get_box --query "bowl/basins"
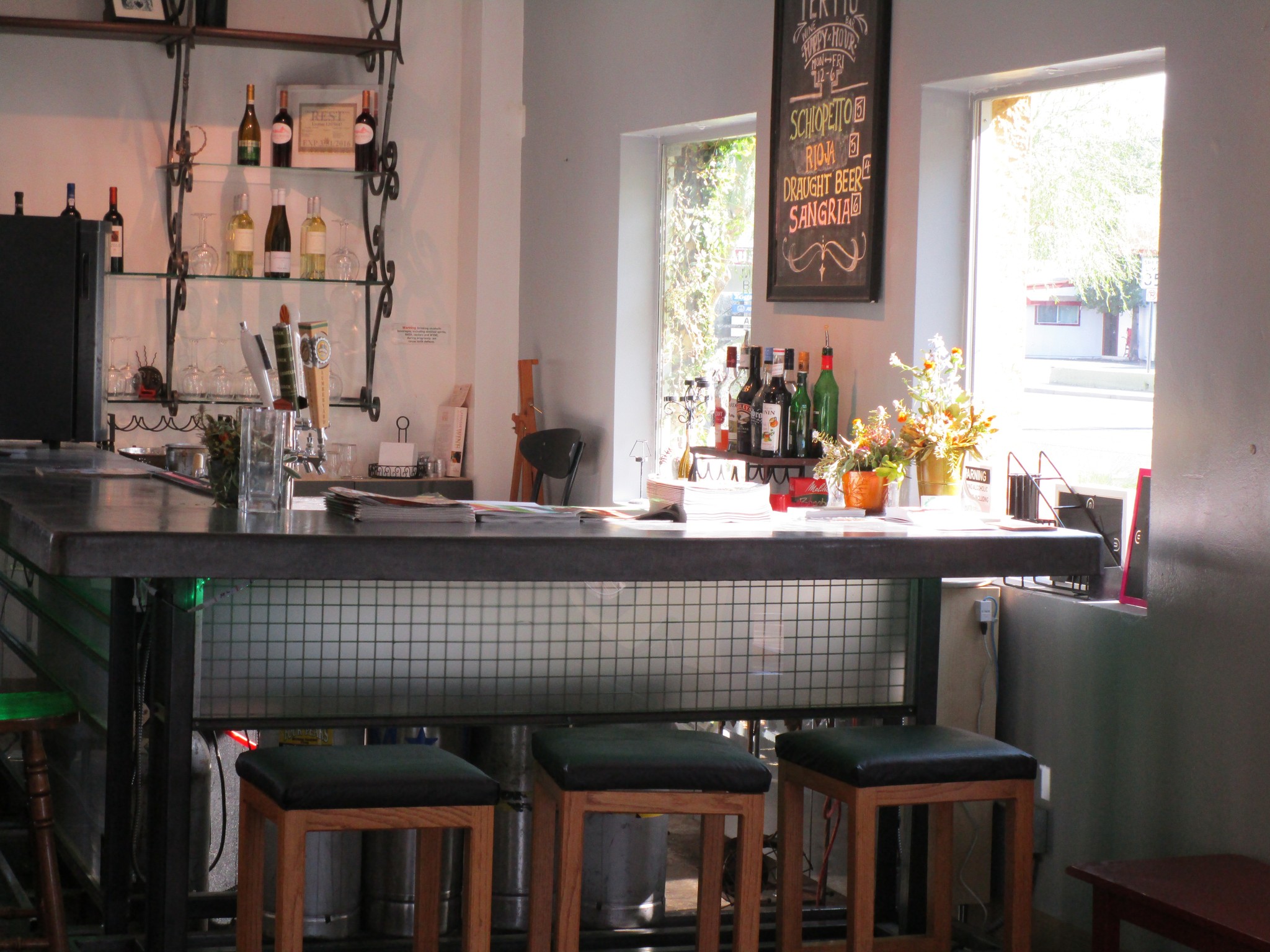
[117,444,211,478]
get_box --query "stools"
[527,723,772,952]
[237,742,499,952]
[0,679,79,952]
[771,725,1038,952]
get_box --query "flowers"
[813,333,998,487]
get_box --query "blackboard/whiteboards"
[765,0,891,303]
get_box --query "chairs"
[518,427,586,505]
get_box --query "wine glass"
[106,333,343,403]
[188,213,219,276]
[327,219,360,280]
[324,442,357,478]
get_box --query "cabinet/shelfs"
[0,1,406,420]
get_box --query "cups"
[427,456,445,479]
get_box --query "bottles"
[226,194,254,276]
[264,187,291,278]
[14,191,23,215]
[195,0,227,28]
[60,183,81,219]
[103,186,123,273]
[272,90,294,167]
[237,84,262,166]
[714,330,839,459]
[354,90,376,172]
[300,197,326,279]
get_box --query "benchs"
[1065,852,1270,952]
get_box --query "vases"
[841,471,890,516]
[916,449,962,498]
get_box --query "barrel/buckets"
[257,723,670,938]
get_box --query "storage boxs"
[434,401,468,477]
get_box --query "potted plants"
[202,414,301,508]
